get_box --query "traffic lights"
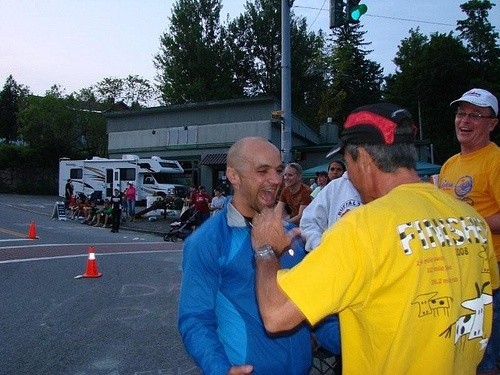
[346,0,367,23]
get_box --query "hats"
[450,88,498,117]
[325,103,417,159]
[129,182,133,185]
[316,171,328,177]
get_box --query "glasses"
[454,111,495,120]
[285,163,301,174]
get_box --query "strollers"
[163,206,203,244]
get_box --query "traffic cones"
[82,245,103,278]
[26,220,40,239]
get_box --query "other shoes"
[69,214,113,228]
[110,228,119,233]
[129,216,136,223]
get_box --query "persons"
[187,185,227,228]
[177,136,341,375]
[64,179,73,210]
[124,182,137,222]
[250,89,500,375]
[67,188,122,232]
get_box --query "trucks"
[59,155,186,205]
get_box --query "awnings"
[199,153,228,165]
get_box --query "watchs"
[254,244,275,258]
